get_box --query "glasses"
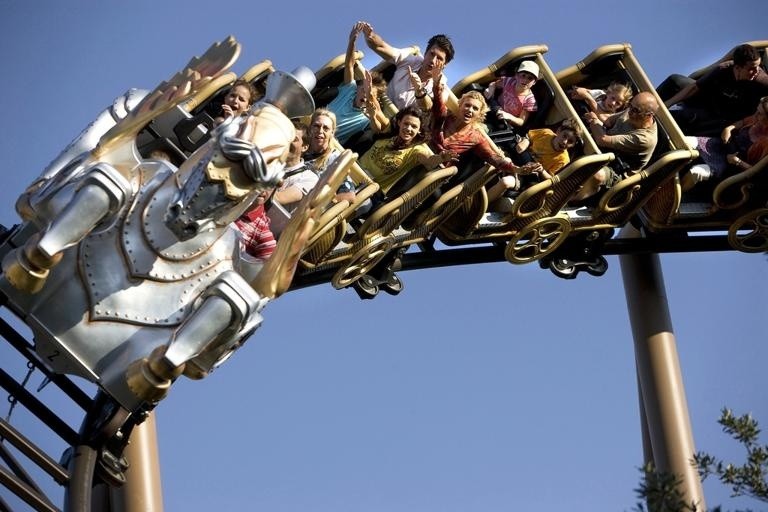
[628,103,650,114]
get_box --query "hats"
[516,60,541,79]
